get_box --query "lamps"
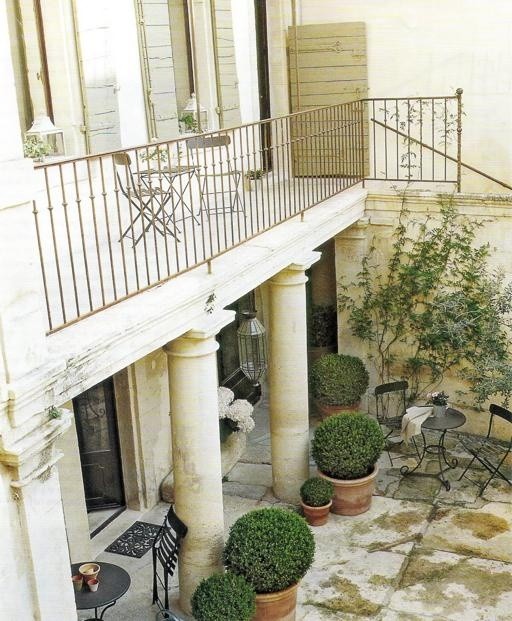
[237,311,268,387]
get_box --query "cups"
[71,563,101,592]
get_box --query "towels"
[400,406,433,446]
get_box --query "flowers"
[428,391,448,404]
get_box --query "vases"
[433,405,447,418]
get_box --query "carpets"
[105,521,172,559]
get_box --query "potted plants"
[311,408,384,516]
[306,307,340,360]
[300,479,336,524]
[222,507,317,621]
[311,350,369,419]
[248,170,264,191]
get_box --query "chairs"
[375,381,421,466]
[112,153,181,248]
[151,504,187,615]
[187,136,247,219]
[457,404,512,497]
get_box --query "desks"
[71,561,131,621]
[134,166,200,233]
[400,407,466,491]
